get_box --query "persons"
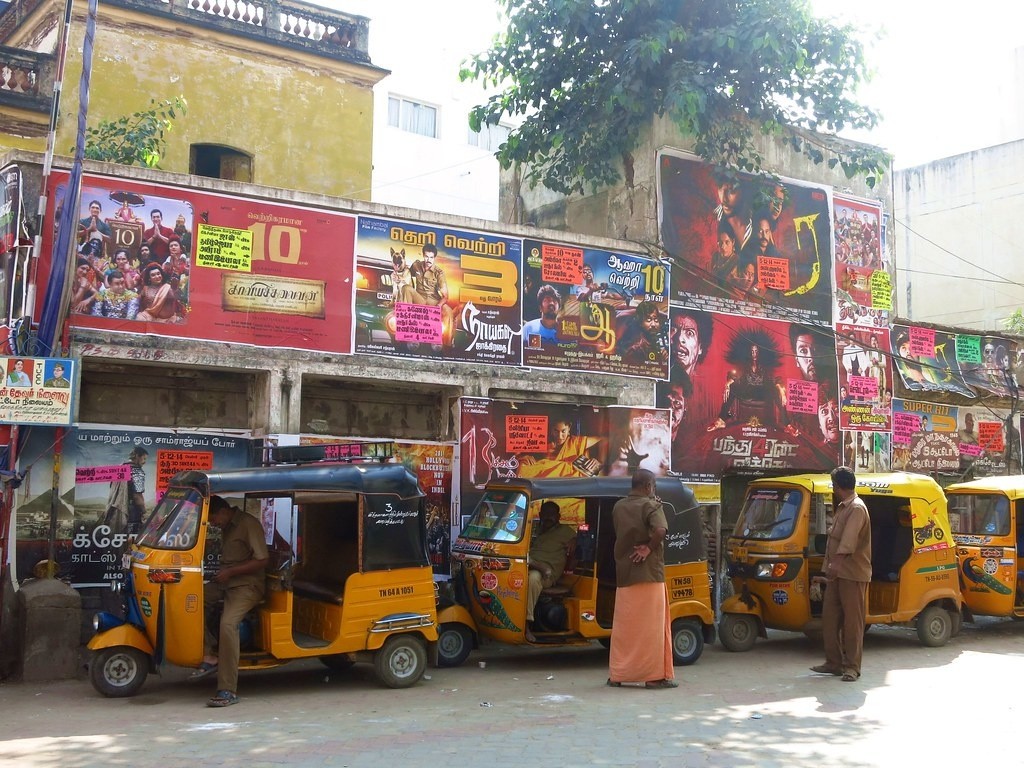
[71,200,189,323]
[841,332,892,403]
[607,469,679,688]
[110,447,148,533]
[710,176,787,304]
[188,495,269,706]
[394,243,453,349]
[958,414,978,444]
[609,411,669,475]
[834,209,880,270]
[526,502,578,642]
[810,467,872,681]
[516,414,608,524]
[894,332,1019,388]
[658,308,840,444]
[843,431,887,468]
[523,262,669,363]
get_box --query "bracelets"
[437,304,440,306]
[80,286,85,289]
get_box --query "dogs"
[389,248,415,306]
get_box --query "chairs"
[534,543,576,633]
[210,545,272,648]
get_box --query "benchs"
[563,566,592,600]
[872,526,914,614]
[293,579,357,643]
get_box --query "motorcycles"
[944,475,1024,627]
[425,473,718,667]
[87,441,439,699]
[716,469,964,651]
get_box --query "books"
[573,455,595,477]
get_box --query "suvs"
[355,255,468,357]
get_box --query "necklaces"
[147,287,159,302]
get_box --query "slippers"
[809,663,844,676]
[206,690,240,706]
[189,661,218,678]
[645,678,679,689]
[842,668,858,681]
[606,677,621,687]
